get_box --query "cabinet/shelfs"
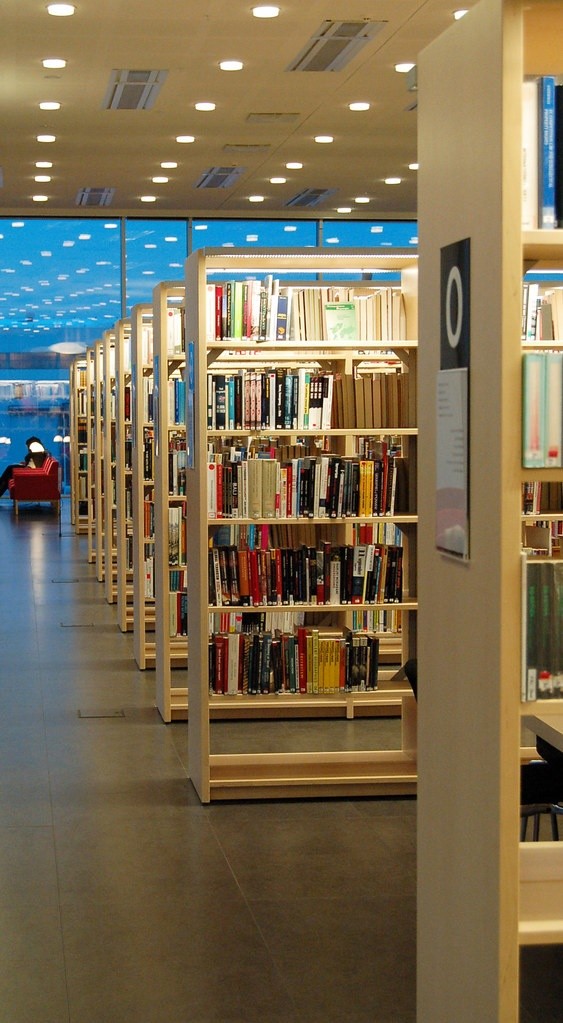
[70,0,563,1023]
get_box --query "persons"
[0,437,46,498]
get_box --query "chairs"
[8,456,61,515]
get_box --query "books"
[521,482,563,704]
[523,281,563,468]
[208,278,409,694]
[524,76,563,228]
[77,301,188,638]
